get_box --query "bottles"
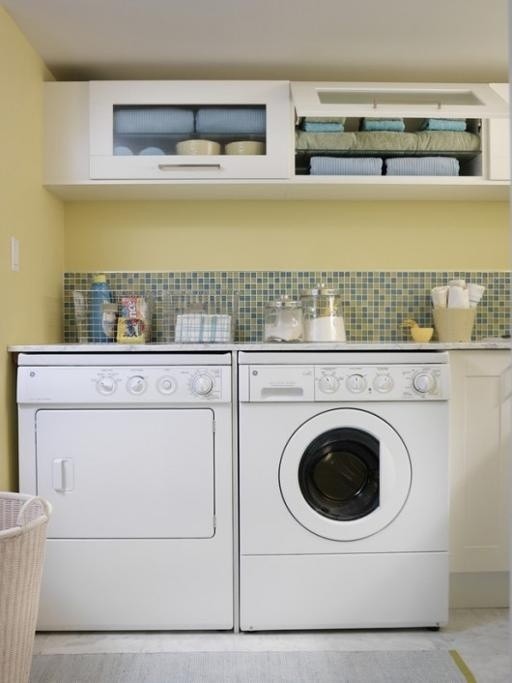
[262,281,346,344]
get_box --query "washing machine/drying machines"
[6,340,238,636]
[236,340,455,633]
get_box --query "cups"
[432,308,476,343]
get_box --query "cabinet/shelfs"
[448,347,511,575]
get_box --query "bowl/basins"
[175,138,265,156]
[410,327,435,343]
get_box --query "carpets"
[28,651,477,683]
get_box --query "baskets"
[0,491,52,683]
